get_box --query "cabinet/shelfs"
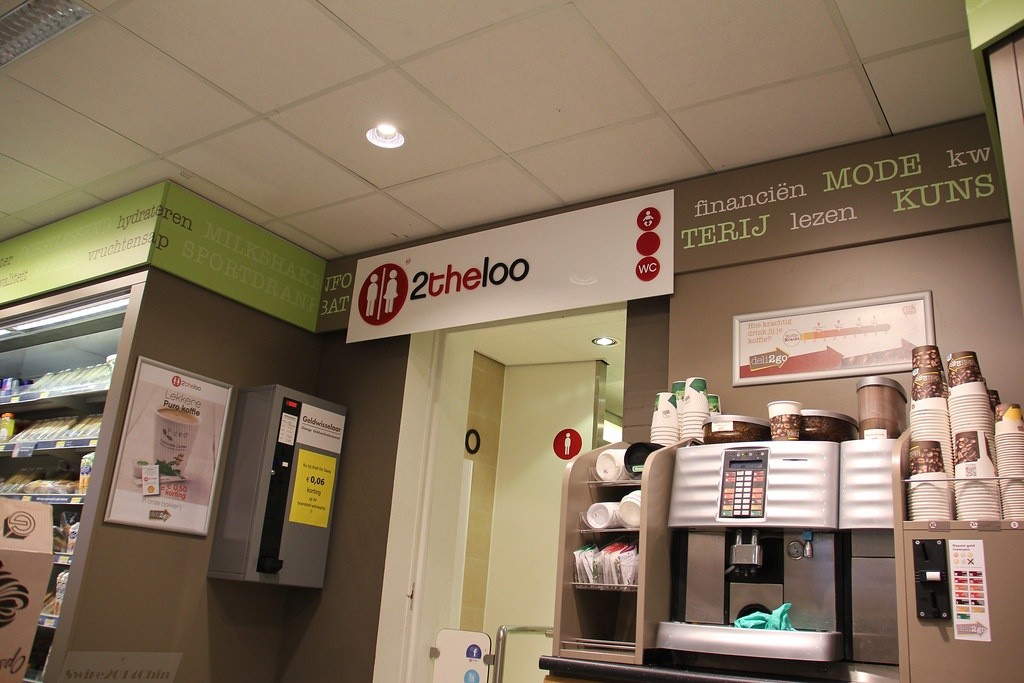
[0,295,132,683]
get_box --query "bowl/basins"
[800,409,859,442]
[702,415,769,443]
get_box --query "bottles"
[0,376,33,402]
[0,413,15,448]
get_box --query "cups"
[907,345,1024,520]
[134,461,149,478]
[105,353,118,377]
[156,408,199,477]
[649,377,722,446]
[767,400,801,441]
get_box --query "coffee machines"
[653,435,900,683]
